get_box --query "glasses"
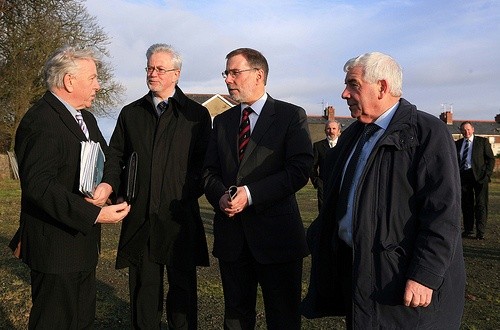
[222,67,258,78]
[144,67,175,74]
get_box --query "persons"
[8,45,130,330]
[310,119,343,215]
[105,44,212,329]
[199,48,316,330]
[454,121,496,241]
[298,51,465,330]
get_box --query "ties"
[75,112,89,141]
[460,140,470,170]
[337,123,380,222]
[238,107,254,164]
[158,101,167,116]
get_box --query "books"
[79,138,105,200]
